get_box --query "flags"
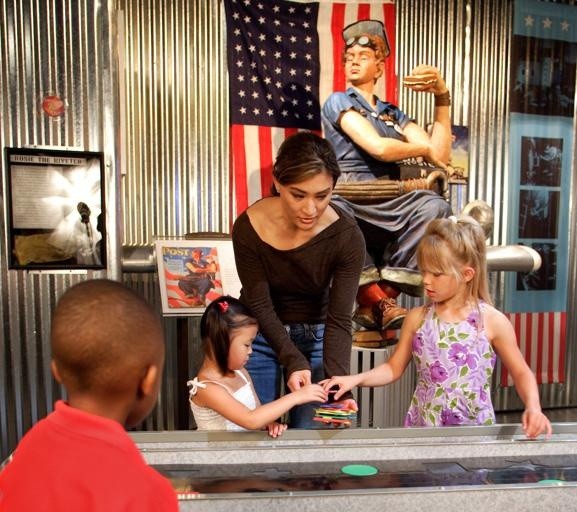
[221,0,399,221]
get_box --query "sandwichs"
[403,73,438,90]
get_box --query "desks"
[121,422,577,512]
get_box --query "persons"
[320,31,459,333]
[0,276,180,511]
[177,248,216,305]
[73,202,100,266]
[314,212,554,439]
[188,296,327,438]
[229,136,367,430]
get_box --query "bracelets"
[432,91,451,107]
